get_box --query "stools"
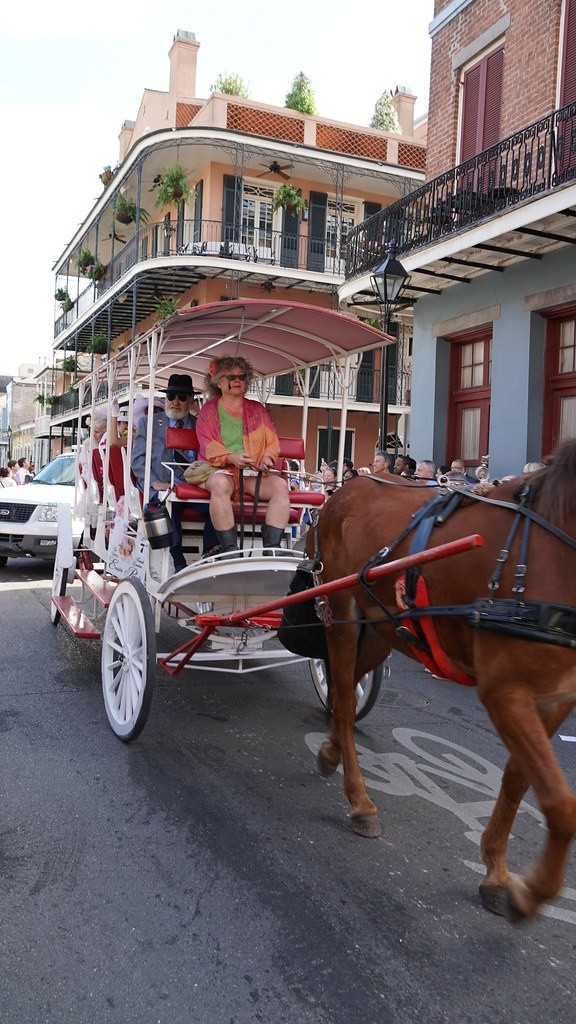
[487,187,519,199]
[425,215,453,229]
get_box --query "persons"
[0,466,17,566]
[524,463,545,472]
[110,394,165,448]
[289,458,370,516]
[7,458,35,485]
[131,374,220,572]
[79,405,108,483]
[372,452,480,485]
[99,406,130,460]
[195,355,294,561]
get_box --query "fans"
[256,160,296,181]
[99,232,127,244]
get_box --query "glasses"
[167,392,187,402]
[218,374,247,380]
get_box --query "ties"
[174,419,189,481]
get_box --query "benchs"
[159,426,327,572]
[98,444,126,511]
[74,454,90,520]
[87,447,103,517]
[122,443,161,521]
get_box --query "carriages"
[47,298,575,922]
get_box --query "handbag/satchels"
[183,461,222,484]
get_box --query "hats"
[133,395,165,428]
[117,406,129,422]
[158,374,201,394]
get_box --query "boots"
[215,524,240,560]
[261,523,294,558]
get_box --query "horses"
[305,436,575,926]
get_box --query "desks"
[438,189,487,215]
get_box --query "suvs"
[0,450,76,568]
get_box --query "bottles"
[143,501,174,550]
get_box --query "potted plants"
[60,354,81,375]
[272,185,307,218]
[32,392,59,406]
[85,332,116,356]
[153,165,200,209]
[112,193,151,225]
[98,164,114,184]
[54,288,74,312]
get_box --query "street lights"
[368,238,412,453]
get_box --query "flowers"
[75,247,108,280]
